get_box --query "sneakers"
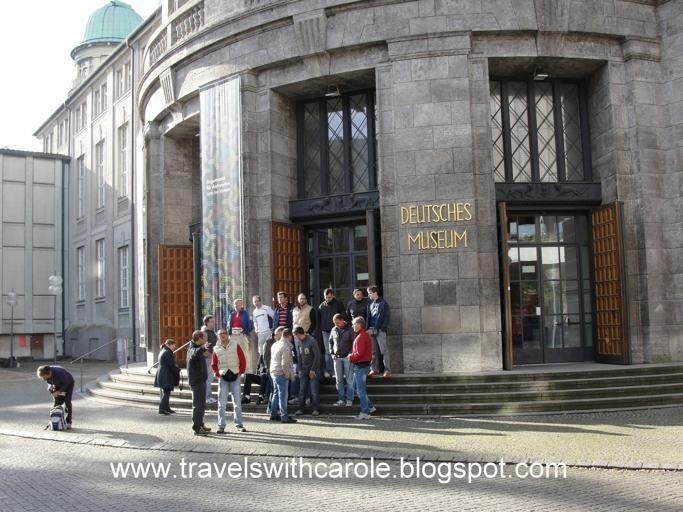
[383,372,390,377]
[324,372,346,378]
[367,370,378,377]
[237,426,245,432]
[66,423,70,429]
[241,397,267,405]
[194,424,223,436]
[209,398,217,403]
[288,399,318,416]
[205,400,213,404]
[267,409,297,422]
[332,400,375,421]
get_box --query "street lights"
[5,287,17,369]
[46,270,63,366]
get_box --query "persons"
[327,310,356,408]
[261,325,285,414]
[251,294,274,358]
[292,326,322,419]
[270,290,296,336]
[36,364,74,430]
[153,337,180,414]
[185,329,211,436]
[239,353,271,404]
[209,327,247,434]
[366,282,392,380]
[268,328,297,424]
[345,286,368,327]
[199,314,217,405]
[290,291,317,406]
[224,298,250,337]
[346,314,378,422]
[316,287,345,380]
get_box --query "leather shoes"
[159,410,169,414]
[167,408,173,413]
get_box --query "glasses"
[218,333,225,335]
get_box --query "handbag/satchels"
[44,405,67,431]
[367,326,378,337]
[174,368,183,391]
